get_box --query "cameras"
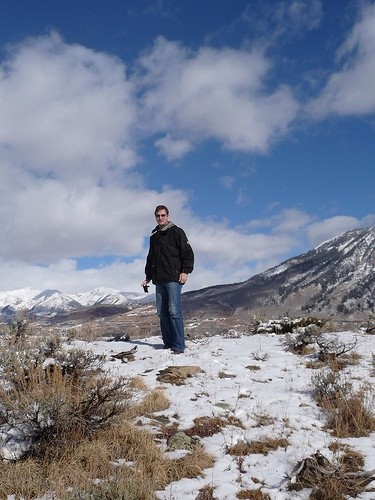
[143,285,149,293]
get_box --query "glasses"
[156,214,167,217]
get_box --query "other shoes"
[171,350,181,354]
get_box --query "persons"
[142,205,194,354]
